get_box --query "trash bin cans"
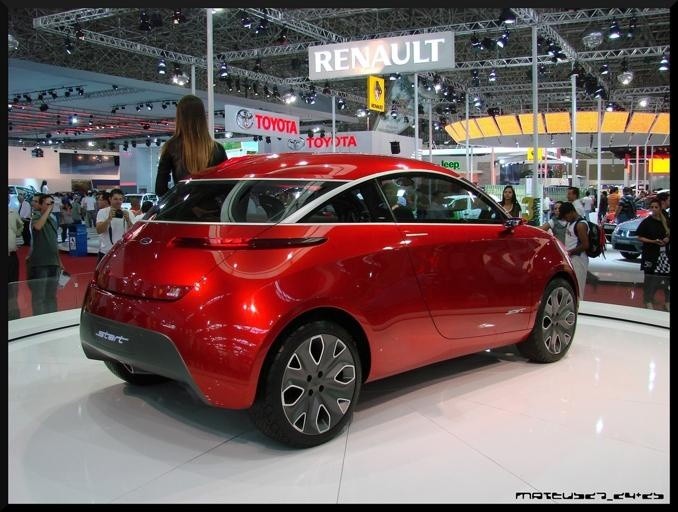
[68,224,88,256]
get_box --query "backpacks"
[575,218,607,258]
[622,202,636,219]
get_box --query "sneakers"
[646,303,654,309]
[663,301,670,310]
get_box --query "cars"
[118,192,158,210]
[602,182,669,259]
[78,151,579,450]
[444,193,502,220]
[7,184,44,213]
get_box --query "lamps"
[659,64,669,71]
[660,52,669,64]
[9,8,638,157]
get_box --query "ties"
[18,204,22,216]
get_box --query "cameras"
[112,209,123,218]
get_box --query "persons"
[155,95,227,197]
[7,173,669,322]
